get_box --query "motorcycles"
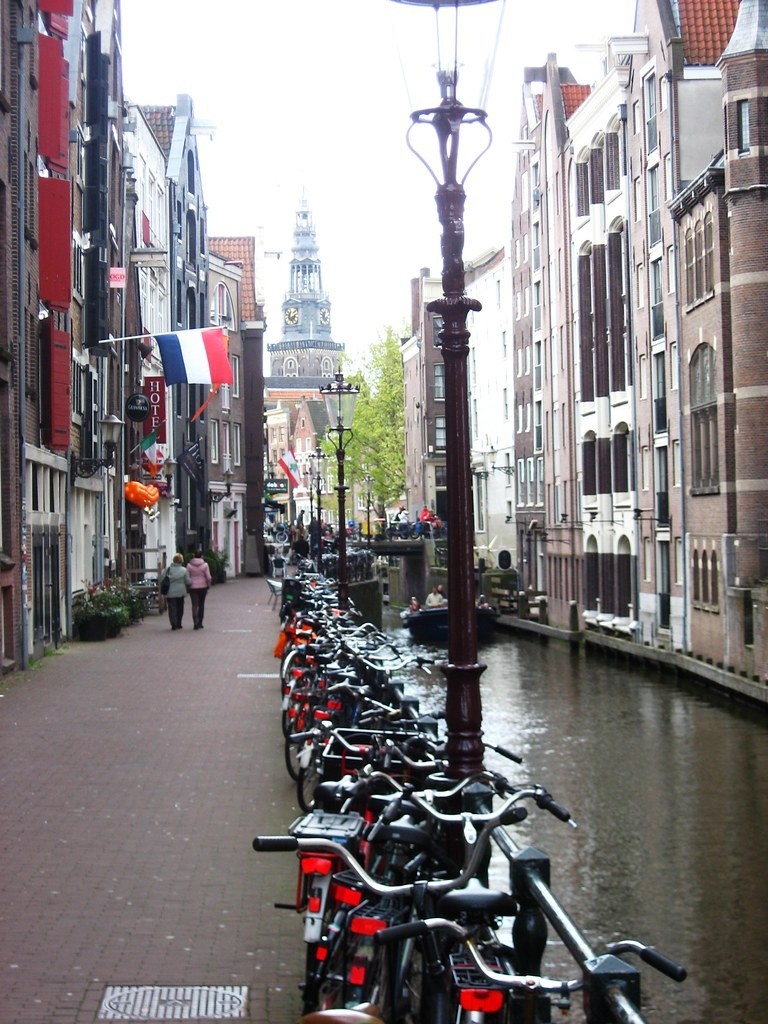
[276,523,291,542]
[386,521,447,539]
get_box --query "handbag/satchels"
[160,567,169,595]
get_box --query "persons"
[296,510,335,538]
[292,535,309,563]
[162,553,192,630]
[477,594,490,609]
[393,506,441,537]
[186,551,212,630]
[409,584,447,613]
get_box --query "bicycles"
[251,559,692,1024]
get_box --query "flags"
[140,427,157,478]
[279,448,301,488]
[153,329,233,387]
[177,440,202,482]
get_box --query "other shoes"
[177,626,183,628]
[198,620,203,628]
[193,621,198,629]
[172,624,177,630]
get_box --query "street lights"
[319,374,361,610]
[361,472,375,542]
[307,446,329,559]
[395,0,493,778]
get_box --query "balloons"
[126,481,159,509]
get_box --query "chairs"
[265,578,282,611]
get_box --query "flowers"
[71,577,149,627]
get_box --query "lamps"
[505,508,675,542]
[158,457,178,492]
[70,414,126,483]
[208,470,234,505]
[138,342,154,357]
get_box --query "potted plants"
[185,549,227,586]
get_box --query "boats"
[403,608,495,648]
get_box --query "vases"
[77,616,121,640]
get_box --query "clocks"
[320,308,330,324]
[284,306,298,325]
